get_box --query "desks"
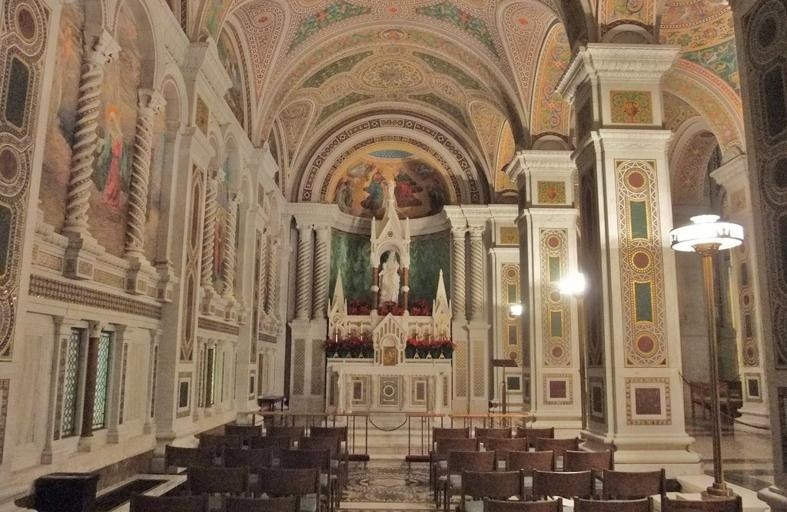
[258,396,285,418]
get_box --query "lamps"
[511,297,528,315]
[559,268,587,300]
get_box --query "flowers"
[324,336,372,350]
[408,336,455,350]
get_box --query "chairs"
[131,425,351,512]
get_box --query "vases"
[325,348,373,358]
[405,348,452,358]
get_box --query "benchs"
[690,382,733,423]
